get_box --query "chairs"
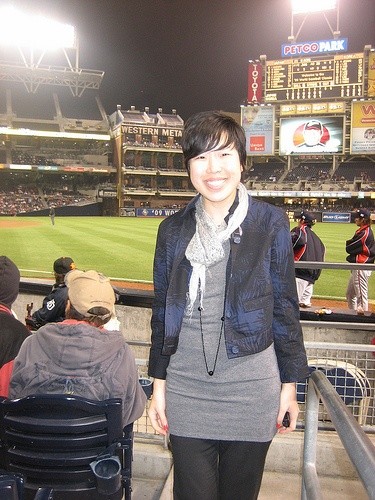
[0,393,131,499]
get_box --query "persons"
[149,110,310,500]
[0,256,32,398]
[33,256,75,331]
[8,269,147,430]
[346,208,375,312]
[291,212,325,306]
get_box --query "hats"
[348,208,371,220]
[0,255,20,305]
[294,211,317,228]
[54,256,75,274]
[64,269,115,319]
